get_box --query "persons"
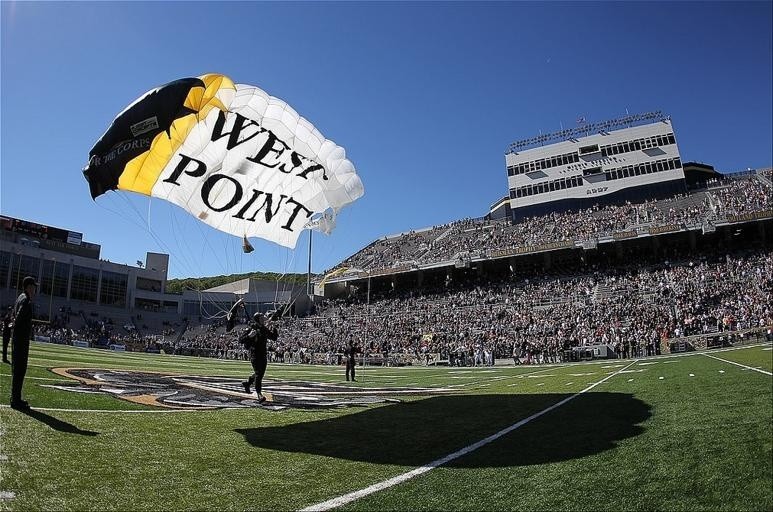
[1,305,13,365]
[311,283,772,364]
[28,308,312,363]
[312,171,771,284]
[235,311,279,404]
[9,274,39,411]
[340,341,364,384]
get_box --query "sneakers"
[9,398,28,409]
[242,381,266,405]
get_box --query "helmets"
[253,312,264,323]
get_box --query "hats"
[22,276,40,288]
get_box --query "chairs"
[0,290,212,343]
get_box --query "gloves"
[247,329,257,339]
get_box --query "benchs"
[345,187,710,272]
